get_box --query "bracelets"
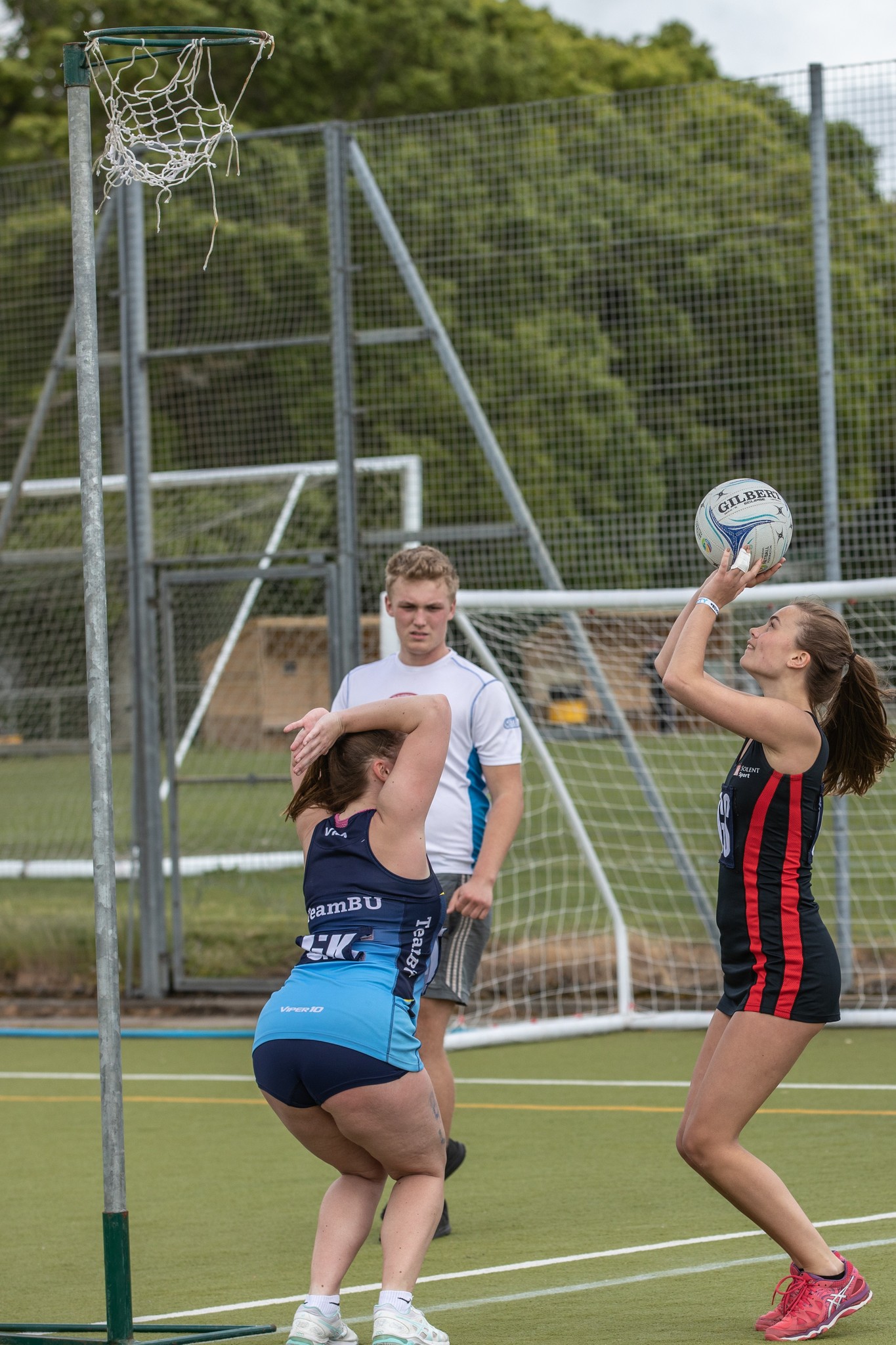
[696,598,719,616]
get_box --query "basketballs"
[694,478,793,575]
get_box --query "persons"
[656,544,896,1345]
[250,695,452,1345]
[330,546,526,1241]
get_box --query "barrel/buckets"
[550,683,591,724]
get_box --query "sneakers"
[285,1305,359,1345]
[755,1250,846,1331]
[371,1304,450,1345]
[765,1261,873,1342]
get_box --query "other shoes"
[380,1200,450,1240]
[444,1137,467,1179]
